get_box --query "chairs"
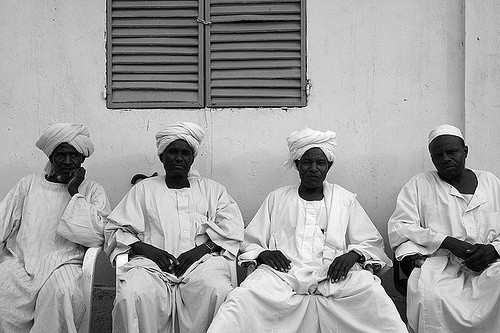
[79,246,408,333]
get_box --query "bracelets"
[349,249,364,264]
[204,242,215,253]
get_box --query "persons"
[205,129,408,333]
[104,122,244,333]
[388,124,500,333]
[0,123,112,333]
